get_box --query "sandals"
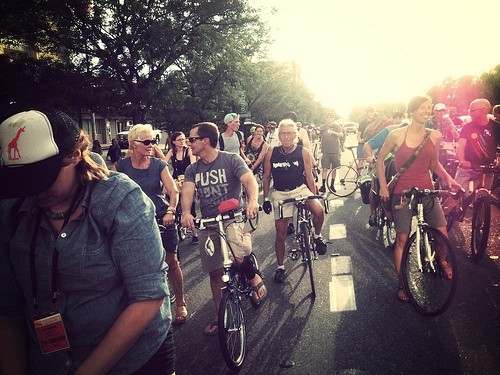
[175,302,187,323]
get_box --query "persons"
[151,131,200,245]
[493,105,500,122]
[449,107,463,125]
[181,122,267,336]
[79,131,107,169]
[218,113,277,175]
[441,99,500,212]
[92,140,102,155]
[112,124,188,321]
[107,139,121,165]
[0,108,175,375]
[263,118,327,282]
[377,95,462,303]
[356,107,410,228]
[317,112,343,193]
[271,111,321,235]
[425,103,459,163]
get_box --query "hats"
[0,108,81,200]
[224,113,240,124]
[250,126,256,134]
[450,107,457,111]
[282,112,298,122]
[433,103,446,112]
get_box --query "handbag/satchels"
[377,176,397,212]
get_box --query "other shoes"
[330,186,336,192]
[369,214,377,226]
[192,235,199,244]
[274,268,285,282]
[288,223,293,234]
[319,186,326,192]
[439,257,454,279]
[397,287,410,301]
[313,237,326,255]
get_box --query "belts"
[274,182,304,191]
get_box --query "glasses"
[136,139,157,145]
[177,139,184,141]
[469,109,481,113]
[188,136,205,141]
[327,117,332,118]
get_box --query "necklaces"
[46,186,86,221]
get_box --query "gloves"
[263,201,272,214]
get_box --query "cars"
[344,123,357,134]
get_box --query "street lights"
[447,94,456,107]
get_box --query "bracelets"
[167,207,176,213]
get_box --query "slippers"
[203,320,219,336]
[251,272,266,302]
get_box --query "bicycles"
[156,192,262,304]
[277,194,327,297]
[362,156,500,317]
[325,145,372,197]
[191,199,265,371]
